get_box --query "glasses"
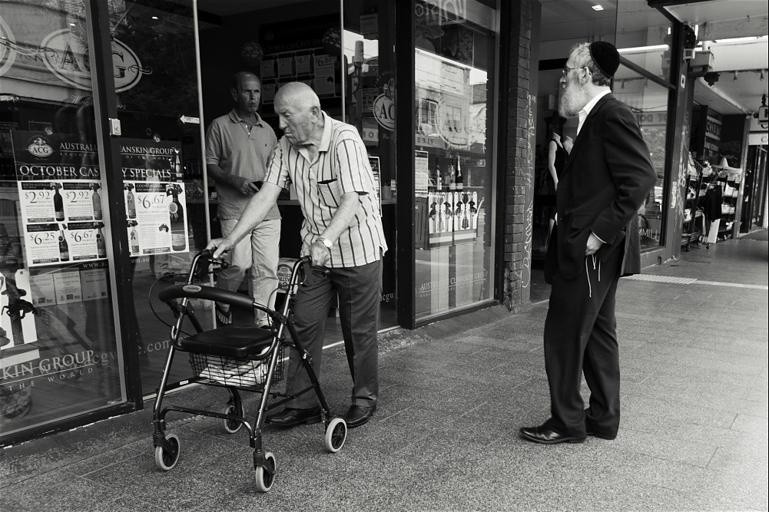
[562,67,583,76]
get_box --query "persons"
[549,131,575,235]
[205,82,389,426]
[205,71,283,382]
[519,39,657,444]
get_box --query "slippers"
[215,301,234,326]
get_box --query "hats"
[588,39,622,79]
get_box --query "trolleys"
[0,253,106,422]
[153,248,348,493]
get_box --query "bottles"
[170,187,186,252]
[58,230,70,263]
[96,224,106,259]
[127,187,137,219]
[433,153,463,192]
[92,184,103,221]
[130,224,139,253]
[53,186,66,221]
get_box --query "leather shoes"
[343,400,377,428]
[583,406,618,440]
[519,418,586,444]
[262,402,323,428]
